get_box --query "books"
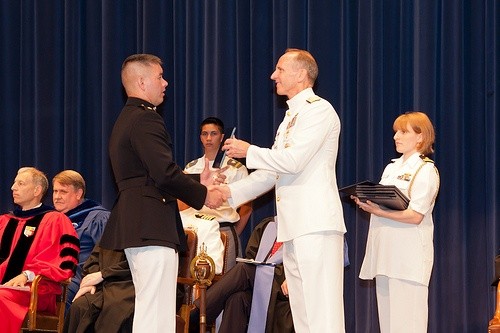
[212,126,236,169]
[338,179,410,210]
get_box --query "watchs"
[24,272,27,276]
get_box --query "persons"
[176,198,225,305]
[62,233,185,333]
[0,167,80,333]
[188,216,295,333]
[183,117,252,274]
[98,53,230,333]
[350,111,441,333]
[52,170,111,318]
[206,48,347,333]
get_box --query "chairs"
[21,274,68,333]
[175,228,228,333]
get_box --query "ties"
[272,241,282,255]
